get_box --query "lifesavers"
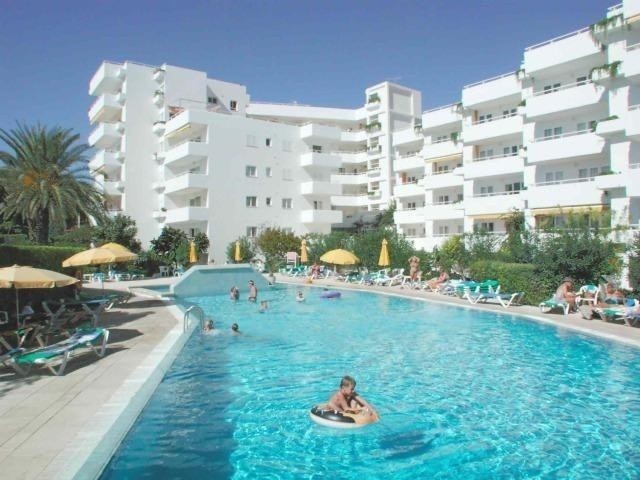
[310,403,378,430]
[320,292,340,298]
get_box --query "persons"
[605,282,624,298]
[247,280,257,303]
[204,319,213,332]
[596,299,640,315]
[431,272,447,284]
[229,323,242,335]
[316,262,322,277]
[260,301,269,313]
[230,285,240,301]
[311,262,316,275]
[322,376,380,422]
[22,299,34,321]
[408,255,420,280]
[296,291,304,302]
[554,276,576,312]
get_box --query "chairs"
[541,284,639,325]
[278,264,423,288]
[0,292,132,376]
[411,275,522,308]
[159,264,182,277]
[83,271,146,281]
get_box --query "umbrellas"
[0,263,81,328]
[320,248,360,264]
[301,239,308,265]
[189,241,197,263]
[61,244,139,295]
[101,241,130,270]
[377,238,391,268]
[235,237,242,264]
